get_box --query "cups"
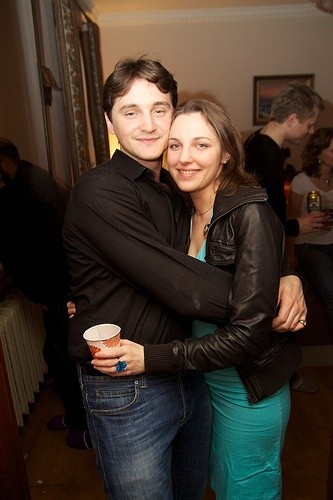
[83,324,121,359]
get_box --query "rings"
[300,320,306,325]
[116,361,127,373]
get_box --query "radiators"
[0,284,49,428]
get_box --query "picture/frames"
[254,73,314,124]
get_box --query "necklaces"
[196,202,214,215]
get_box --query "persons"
[289,128,333,345]
[243,83,326,394]
[62,53,308,500]
[68,99,307,500]
[0,138,93,449]
